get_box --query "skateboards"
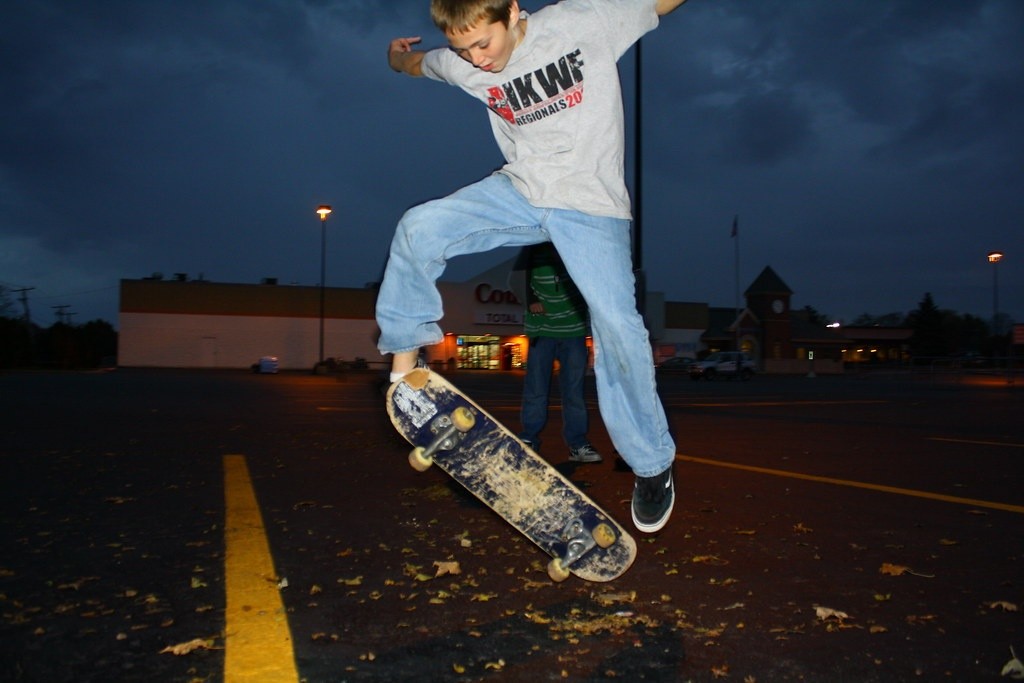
[386,368,638,583]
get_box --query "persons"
[506,232,610,466]
[374,1,679,533]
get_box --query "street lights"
[986,251,1005,374]
[315,204,333,359]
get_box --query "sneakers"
[569,444,601,462]
[632,462,677,532]
[522,439,535,450]
[384,357,431,395]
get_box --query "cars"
[252,356,280,374]
[655,356,697,374]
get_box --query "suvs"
[687,350,760,383]
